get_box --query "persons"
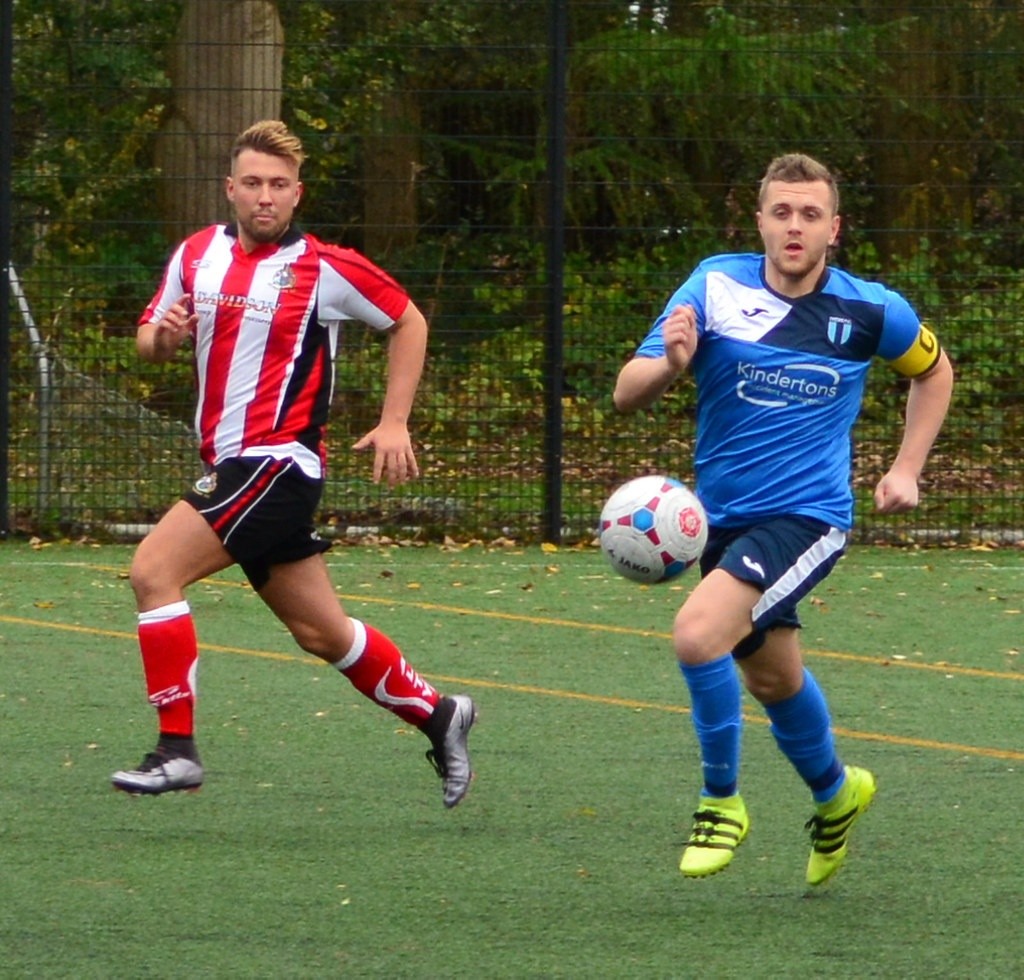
[112,118,476,808]
[614,153,957,885]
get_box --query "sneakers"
[110,734,204,796]
[419,694,476,807]
[805,764,877,886]
[679,791,749,879]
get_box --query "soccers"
[598,475,707,584]
